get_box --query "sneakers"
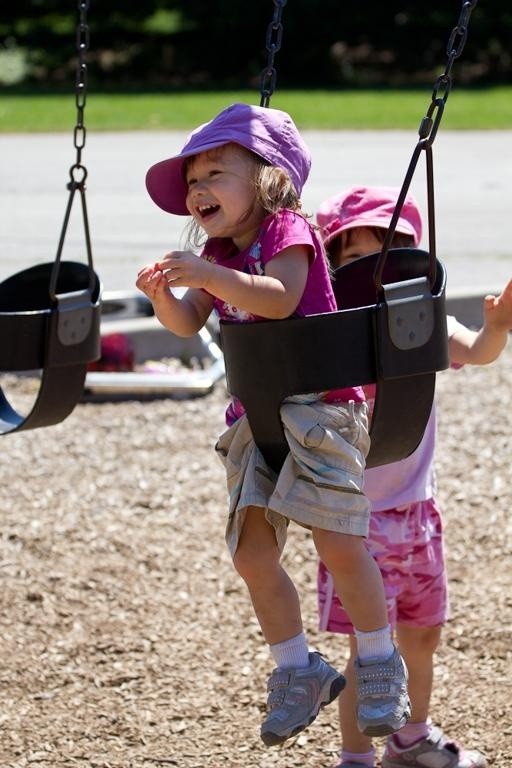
[353,641,413,737]
[381,725,487,768]
[260,652,347,747]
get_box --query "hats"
[145,103,313,218]
[314,188,423,251]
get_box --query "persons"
[271,185,510,766]
[129,104,415,745]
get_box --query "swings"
[219,1,477,479]
[1,1,102,436]
